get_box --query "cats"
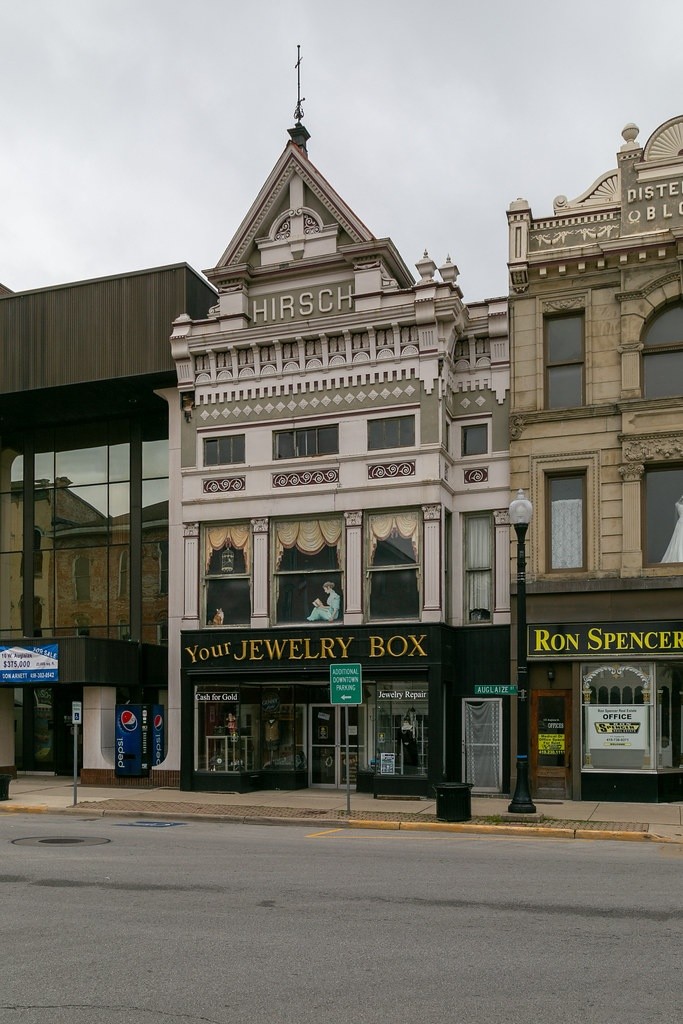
[214,608,224,625]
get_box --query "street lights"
[507,488,538,814]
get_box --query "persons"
[263,719,278,766]
[396,721,421,775]
[660,495,683,563]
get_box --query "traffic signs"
[330,664,363,704]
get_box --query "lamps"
[547,665,556,688]
